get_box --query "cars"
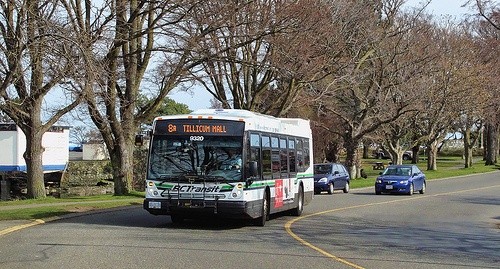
[314,163,350,194]
[376,147,413,160]
[374,164,427,196]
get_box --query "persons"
[220,150,242,170]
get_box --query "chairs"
[396,168,401,174]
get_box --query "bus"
[134,109,313,227]
